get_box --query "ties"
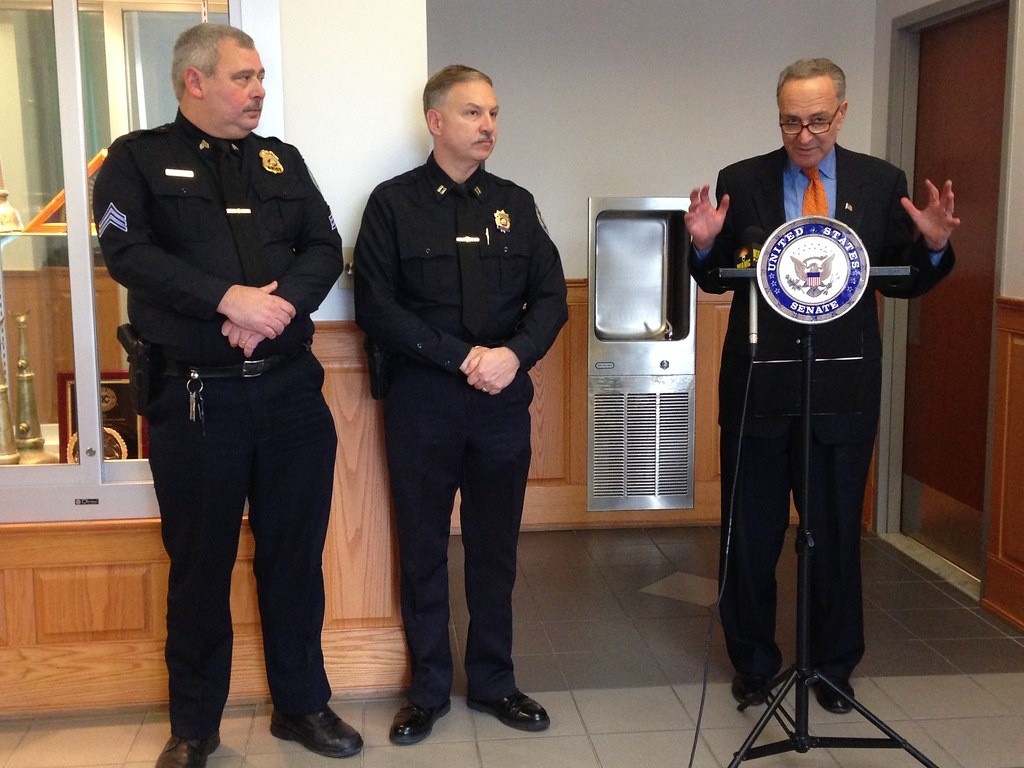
[454,183,493,337]
[219,141,269,287]
[800,166,828,218]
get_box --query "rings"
[483,387,488,392]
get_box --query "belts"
[158,356,280,384]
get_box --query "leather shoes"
[730,651,782,705]
[466,691,550,731]
[154,729,220,768]
[388,699,451,744]
[815,680,856,712]
[270,707,364,757]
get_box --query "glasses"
[779,106,840,134]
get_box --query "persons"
[352,60,571,745]
[92,23,364,767]
[683,53,961,714]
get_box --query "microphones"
[732,225,765,358]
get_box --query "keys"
[188,391,206,436]
